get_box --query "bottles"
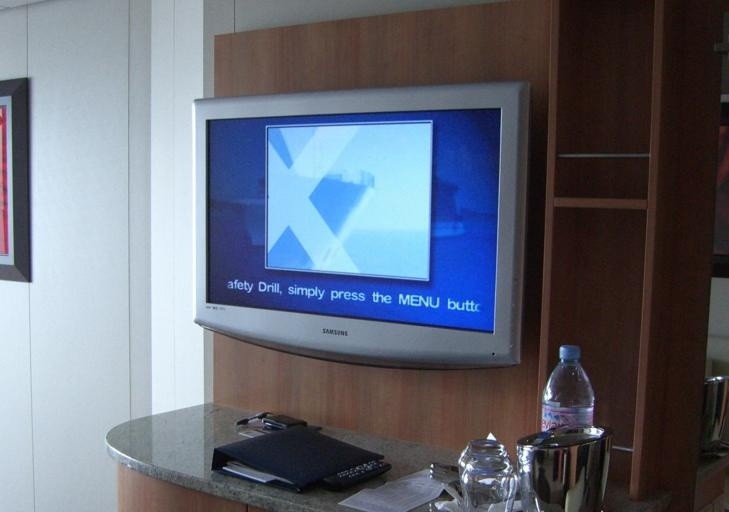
[541,346,595,432]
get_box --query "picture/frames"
[0,78,34,283]
[710,104,728,277]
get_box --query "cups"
[458,439,515,512]
[460,454,517,512]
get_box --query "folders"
[212,424,384,494]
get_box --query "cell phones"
[261,414,308,432]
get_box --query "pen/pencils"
[236,412,268,425]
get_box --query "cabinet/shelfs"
[540,1,664,499]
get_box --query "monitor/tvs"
[192,80,532,371]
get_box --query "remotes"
[322,459,393,492]
